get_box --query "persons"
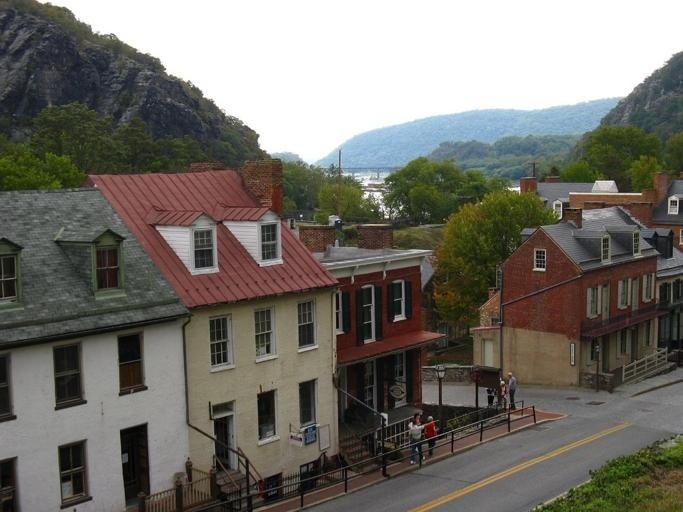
[408,413,439,465]
[487,372,516,412]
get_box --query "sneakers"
[423,456,425,460]
[410,461,416,464]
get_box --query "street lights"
[595,343,601,393]
[435,365,447,438]
